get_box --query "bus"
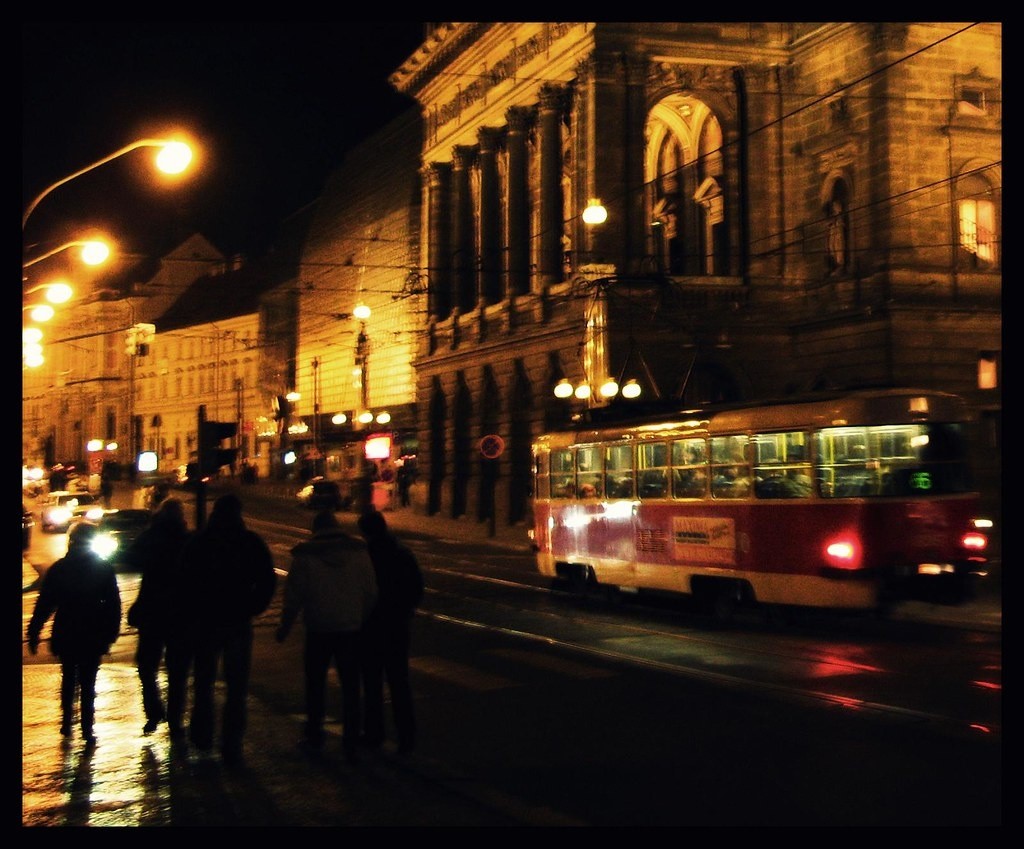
[527,386,1000,629]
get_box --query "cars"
[22,505,40,550]
[40,489,107,532]
[98,508,154,564]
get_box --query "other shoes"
[387,750,418,770]
[144,711,165,734]
[83,733,97,742]
[61,725,71,735]
[298,737,323,756]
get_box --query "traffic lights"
[124,321,157,362]
[196,401,239,476]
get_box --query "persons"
[273,507,379,753]
[126,495,279,763]
[398,459,416,507]
[355,511,425,756]
[550,435,933,499]
[26,524,120,743]
